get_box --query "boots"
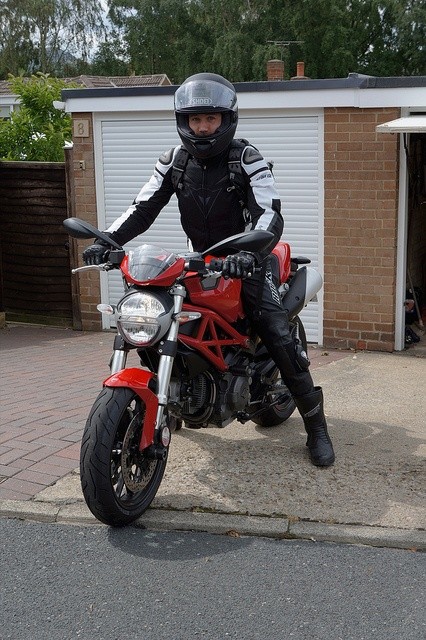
[292,386,335,467]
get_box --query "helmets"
[173,72,239,161]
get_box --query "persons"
[81,72,335,466]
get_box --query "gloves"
[221,252,256,281]
[82,241,110,266]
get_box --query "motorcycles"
[61,215,323,527]
